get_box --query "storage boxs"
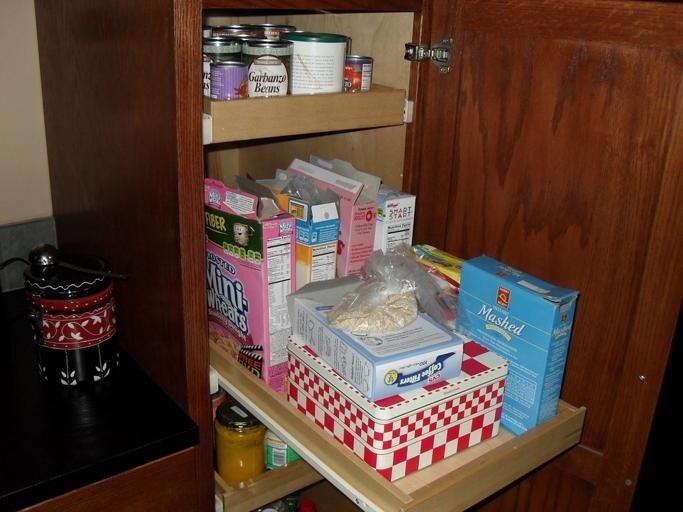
[409,242,463,315]
[205,160,417,390]
[453,253,580,439]
[287,282,508,482]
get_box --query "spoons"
[27,241,131,282]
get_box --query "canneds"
[345,55,373,92]
[214,398,267,488]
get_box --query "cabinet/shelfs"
[33,0,683,511]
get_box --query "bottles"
[205,382,298,485]
[20,252,122,396]
